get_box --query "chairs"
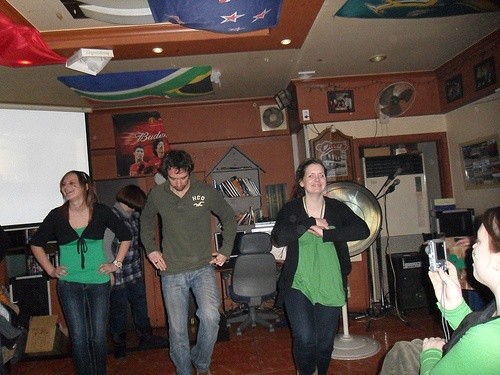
[226,232,280,336]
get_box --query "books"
[215,205,260,228]
[213,176,261,198]
[27,250,59,275]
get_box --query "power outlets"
[303,110,310,121]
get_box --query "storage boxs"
[430,208,477,239]
[25,315,68,358]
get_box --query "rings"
[155,261,158,264]
[216,258,218,261]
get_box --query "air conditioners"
[361,152,430,308]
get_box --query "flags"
[333,0,500,19]
[56,64,215,105]
[147,0,283,34]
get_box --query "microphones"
[384,167,402,186]
[387,179,400,192]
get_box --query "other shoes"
[192,365,210,375]
[113,343,126,359]
[142,337,165,347]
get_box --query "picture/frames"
[473,55,496,92]
[444,73,464,104]
[327,90,355,113]
[456,132,500,190]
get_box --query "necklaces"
[304,195,325,219]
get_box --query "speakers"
[386,251,436,314]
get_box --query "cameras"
[425,239,448,272]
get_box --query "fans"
[323,181,382,360]
[374,81,416,124]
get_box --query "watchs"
[112,259,123,269]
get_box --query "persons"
[141,150,238,375]
[0,225,28,375]
[104,184,169,359]
[379,206,500,375]
[453,236,477,247]
[271,157,371,375]
[29,170,133,375]
[148,138,166,176]
[129,145,157,177]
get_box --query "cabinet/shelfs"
[205,144,266,230]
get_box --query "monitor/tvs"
[215,231,246,258]
